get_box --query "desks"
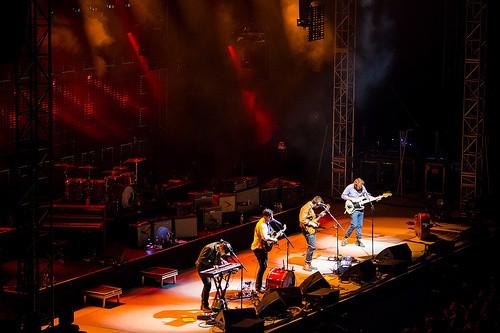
[141,268,177,287]
[81,285,122,308]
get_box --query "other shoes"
[201,305,212,313]
[356,239,366,247]
[256,289,265,294]
[303,262,312,271]
[341,237,347,246]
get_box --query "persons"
[195,241,230,313]
[341,178,381,247]
[299,196,326,271]
[251,208,284,294]
[402,271,500,333]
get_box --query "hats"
[222,241,231,255]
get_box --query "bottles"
[239,214,244,224]
[146,233,179,250]
[274,203,282,212]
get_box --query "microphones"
[272,218,281,225]
[318,202,326,206]
[358,191,366,197]
[220,239,230,246]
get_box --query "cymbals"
[113,167,129,170]
[78,166,97,169]
[54,164,77,168]
[125,158,146,162]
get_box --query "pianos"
[199,263,242,315]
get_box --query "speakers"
[340,243,412,283]
[214,271,339,333]
[126,177,303,249]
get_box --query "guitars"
[345,191,392,215]
[262,224,287,252]
[301,204,330,235]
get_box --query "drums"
[90,180,108,201]
[266,267,295,289]
[121,172,136,184]
[111,184,135,209]
[72,178,87,200]
[104,175,122,183]
